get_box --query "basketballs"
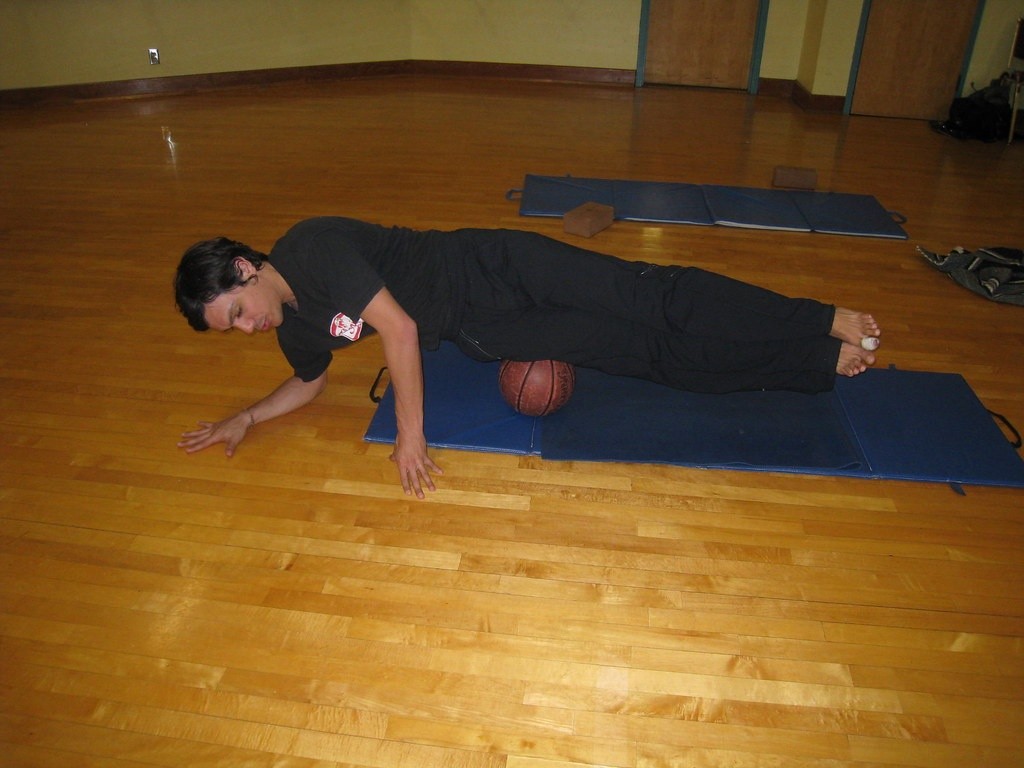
[497,358,577,417]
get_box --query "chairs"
[1001,17,1024,146]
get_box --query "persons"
[174,217,879,499]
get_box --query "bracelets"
[246,409,254,427]
